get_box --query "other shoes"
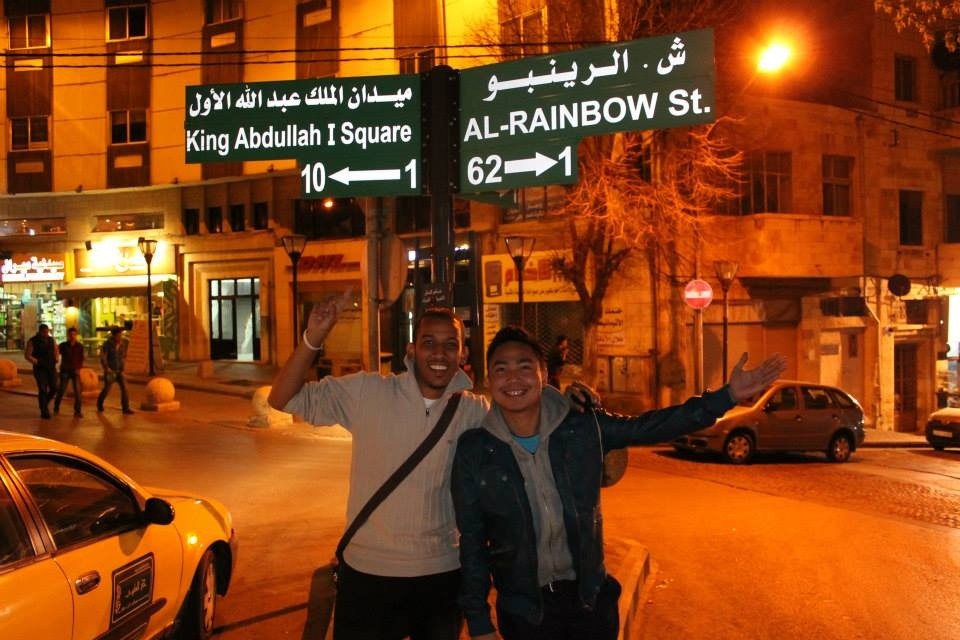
[40,411,51,418]
[74,405,81,413]
[53,400,58,411]
[96,402,104,412]
[122,408,135,415]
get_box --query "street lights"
[279,234,306,352]
[503,235,537,329]
[137,236,158,377]
[711,258,739,387]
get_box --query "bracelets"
[303,329,324,352]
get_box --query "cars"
[0,426,240,640]
[669,377,866,466]
[923,396,960,453]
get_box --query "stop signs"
[684,278,713,309]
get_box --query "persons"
[448,326,788,640]
[23,324,60,419]
[267,299,601,640]
[95,327,138,414]
[54,327,87,418]
[546,335,570,390]
